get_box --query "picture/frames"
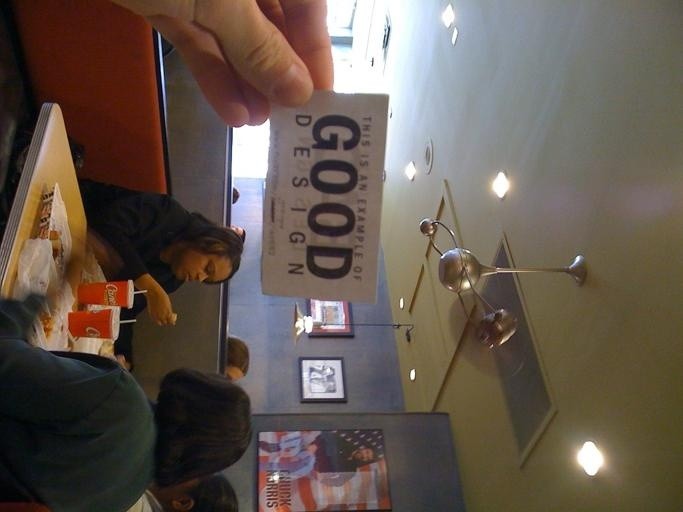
[304,298,354,338]
[255,429,392,512]
[297,356,347,404]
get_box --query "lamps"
[292,301,415,347]
[419,218,587,351]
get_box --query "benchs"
[0,0,171,197]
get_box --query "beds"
[0,102,114,367]
